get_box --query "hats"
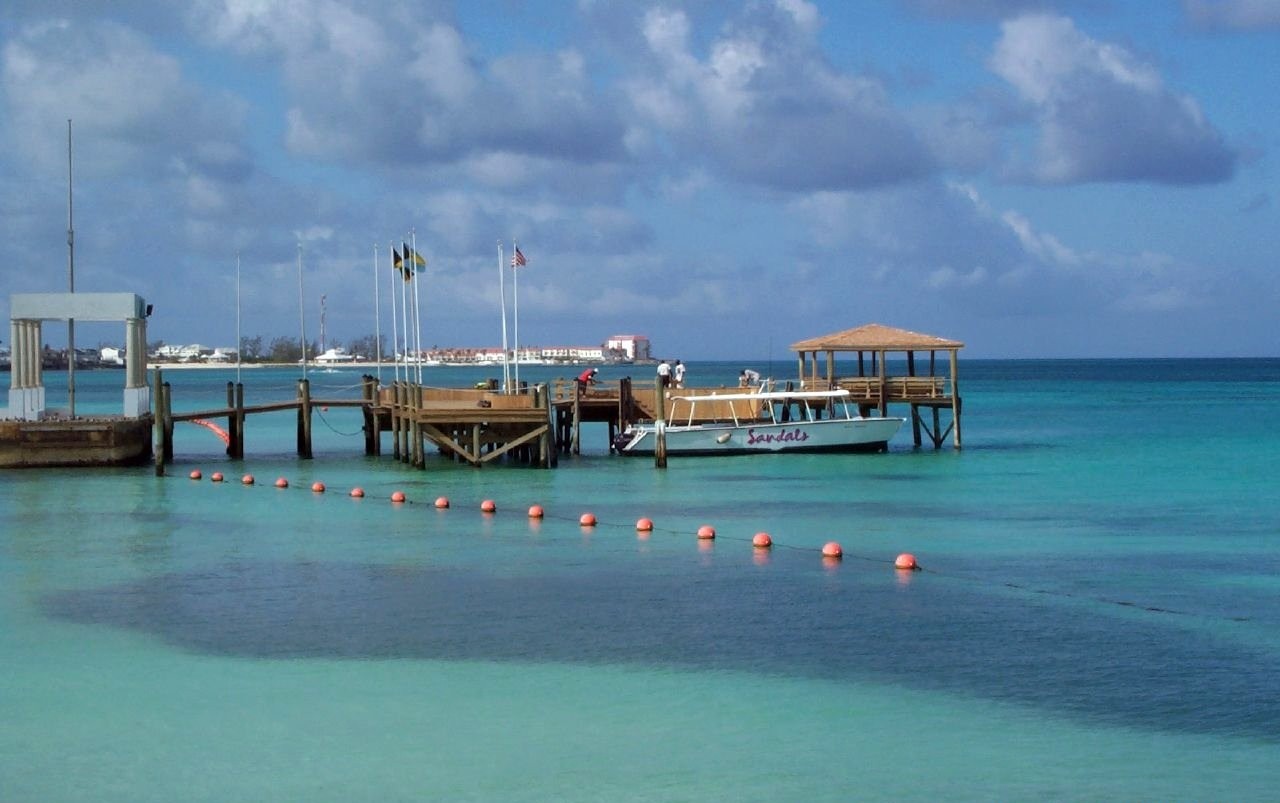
[594,368,598,372]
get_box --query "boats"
[609,378,908,458]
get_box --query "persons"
[738,369,761,388]
[657,359,685,389]
[578,368,600,398]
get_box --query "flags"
[511,247,526,268]
[393,242,425,283]
